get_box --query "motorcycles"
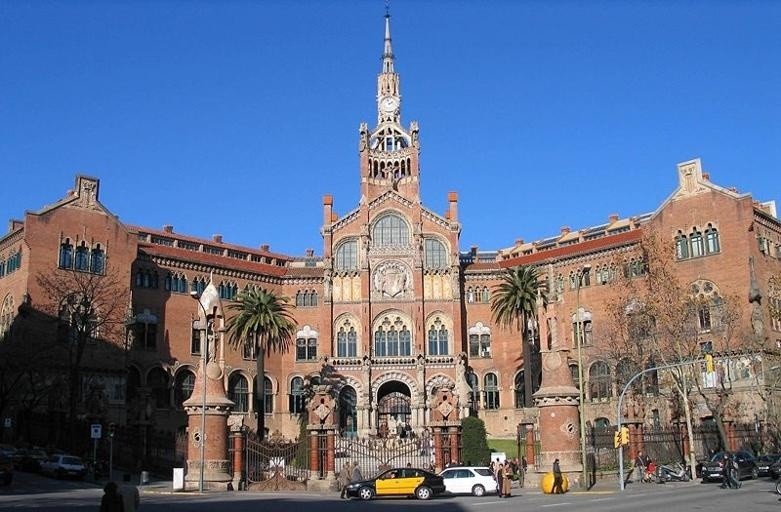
[656,456,692,482]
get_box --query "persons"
[634,451,655,484]
[340,461,363,499]
[489,456,527,498]
[100,473,140,512]
[207,314,217,363]
[390,472,397,479]
[719,453,744,489]
[550,459,564,494]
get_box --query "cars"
[435,466,501,497]
[341,467,446,501]
[700,450,781,482]
[0,442,85,486]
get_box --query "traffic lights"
[614,425,631,449]
[706,354,718,375]
[108,421,116,438]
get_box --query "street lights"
[189,290,208,493]
[577,264,593,490]
